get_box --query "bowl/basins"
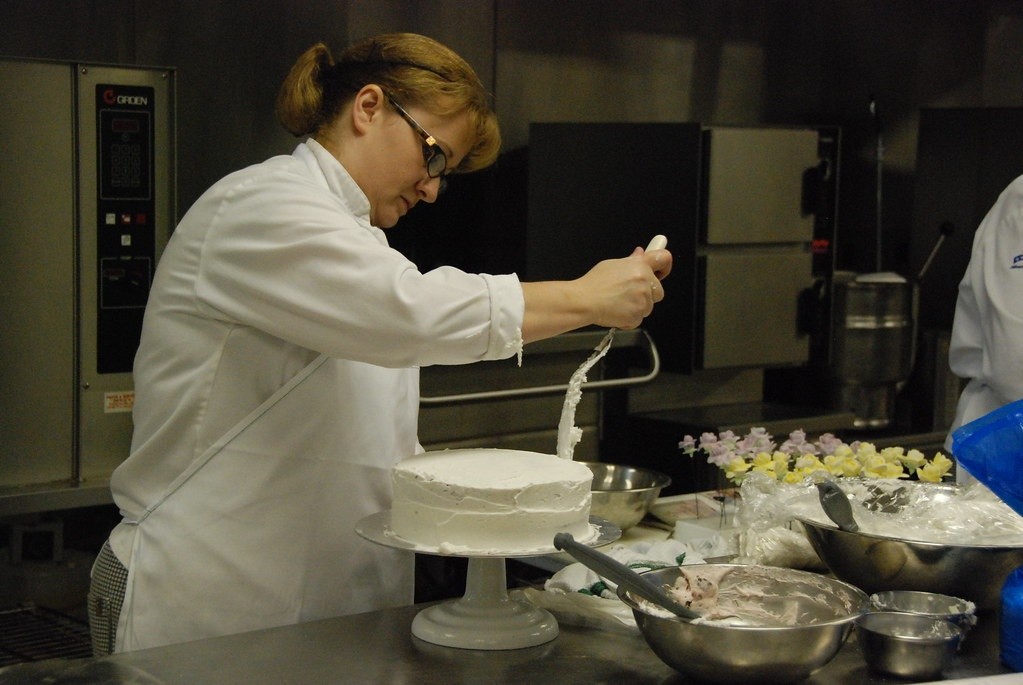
[794,479,1023,612]
[616,564,870,684]
[578,461,673,533]
[855,612,962,679]
[869,590,974,648]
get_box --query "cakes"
[391,448,594,547]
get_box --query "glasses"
[353,82,457,195]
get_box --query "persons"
[941,173,1023,484]
[85,31,672,659]
